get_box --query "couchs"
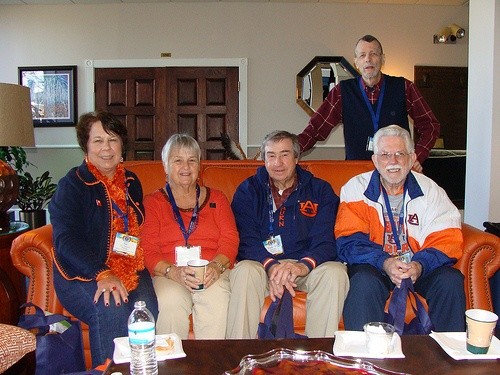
[11,160,500,334]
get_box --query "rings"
[184,275,187,279]
[111,287,117,292]
[102,288,109,292]
[286,268,291,274]
[210,279,214,282]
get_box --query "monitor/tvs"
[414,66,469,150]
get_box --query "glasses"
[375,152,411,160]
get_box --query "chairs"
[0,266,36,375]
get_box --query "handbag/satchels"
[15,302,86,374]
[382,277,432,336]
[256,288,308,340]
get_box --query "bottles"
[126,300,158,375]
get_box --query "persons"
[47,110,159,370]
[140,134,240,342]
[223,129,351,340]
[332,124,467,333]
[296,34,440,174]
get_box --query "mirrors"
[295,55,359,118]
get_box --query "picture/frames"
[18,64,79,127]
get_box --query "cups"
[464,308,500,355]
[363,321,395,356]
[187,259,210,291]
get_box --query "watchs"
[164,264,175,277]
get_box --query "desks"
[0,222,30,303]
[101,338,500,375]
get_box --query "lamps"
[433,22,465,43]
[0,82,36,229]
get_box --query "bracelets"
[208,260,226,273]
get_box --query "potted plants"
[14,168,57,229]
